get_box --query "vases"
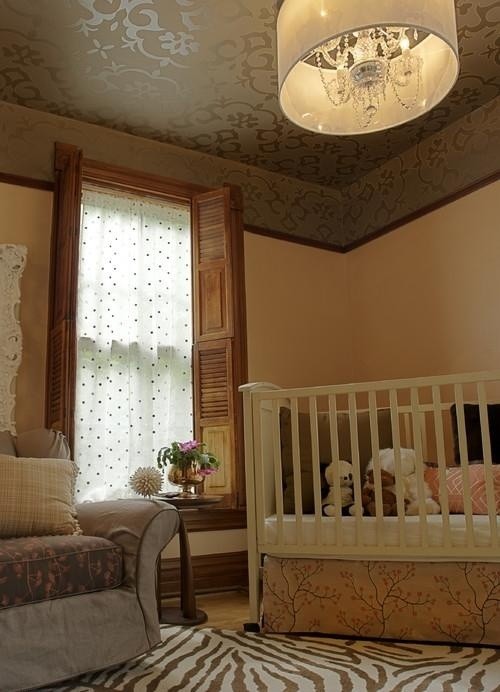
[165,463,206,499]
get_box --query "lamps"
[276,1,461,137]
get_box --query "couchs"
[0,428,181,692]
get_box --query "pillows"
[0,430,17,457]
[450,403,500,466]
[15,429,71,460]
[0,454,83,539]
[423,463,500,516]
[279,406,393,515]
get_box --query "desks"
[154,504,247,626]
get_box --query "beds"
[238,380,500,649]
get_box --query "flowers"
[156,440,222,480]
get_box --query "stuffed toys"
[322,460,364,515]
[367,445,441,516]
[364,468,411,517]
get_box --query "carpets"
[21,622,500,692]
